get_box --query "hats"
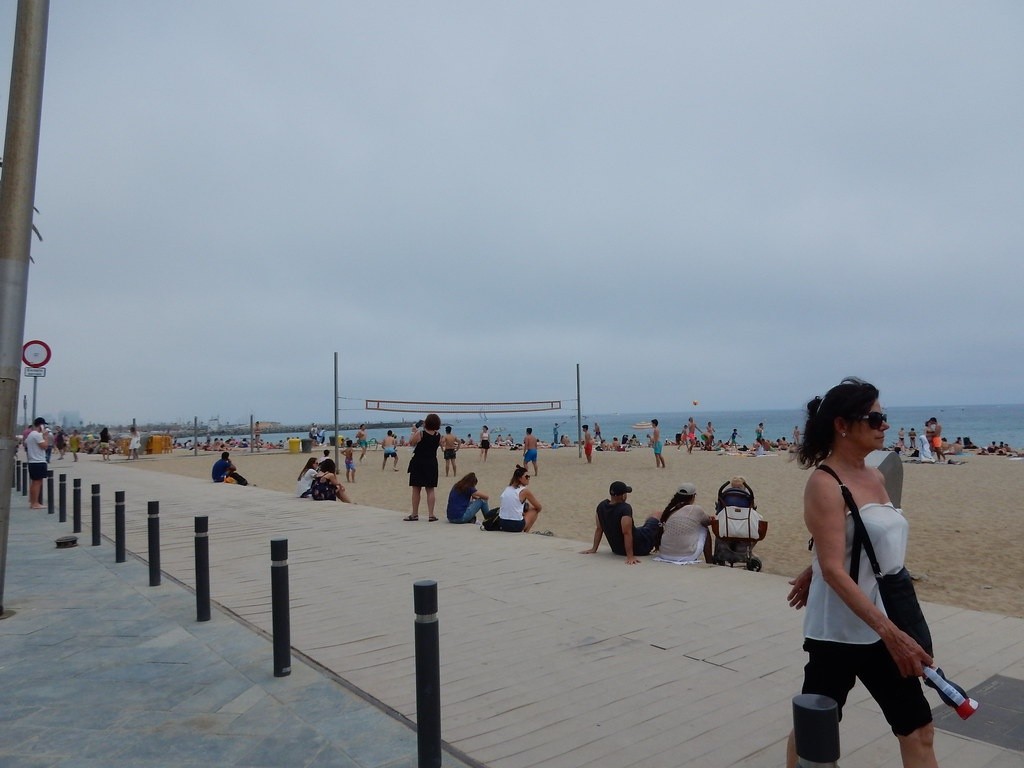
[678,483,696,496]
[34,417,48,424]
[45,428,51,432]
[609,480,632,496]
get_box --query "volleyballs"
[693,400,698,405]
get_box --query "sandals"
[403,515,419,521]
[429,516,438,522]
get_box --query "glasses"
[522,474,530,479]
[859,411,889,432]
[312,462,318,465]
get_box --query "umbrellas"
[83,434,97,440]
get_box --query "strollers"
[711,481,768,572]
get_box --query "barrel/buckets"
[338,436,344,447]
[329,436,336,446]
[289,439,300,454]
[676,434,681,444]
[301,439,312,453]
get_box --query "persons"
[580,482,664,564]
[21,426,112,461]
[319,450,329,462]
[786,376,939,768]
[212,452,236,483]
[315,459,356,504]
[403,414,442,521]
[499,464,542,533]
[341,440,356,483]
[883,417,1024,462]
[447,472,489,524]
[382,430,399,471]
[731,477,746,491]
[127,427,140,460]
[356,423,367,462]
[24,417,47,509]
[297,457,319,498]
[658,483,715,565]
[172,421,325,452]
[392,417,800,477]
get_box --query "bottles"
[922,663,979,720]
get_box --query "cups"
[224,475,226,483]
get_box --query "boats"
[632,422,653,428]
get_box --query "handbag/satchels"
[714,496,762,540]
[407,457,413,474]
[479,507,501,531]
[223,472,248,486]
[876,565,934,665]
